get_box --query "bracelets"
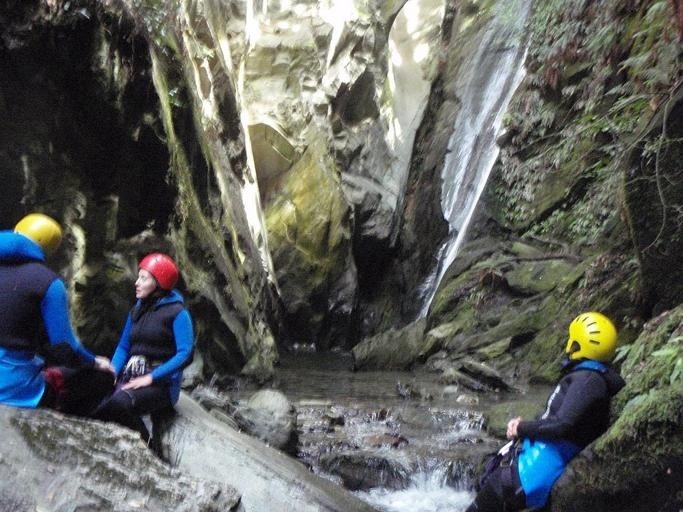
[506,416,521,440]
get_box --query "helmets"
[15,213,63,260]
[139,253,179,291]
[566,311,617,361]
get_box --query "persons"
[0,213,116,417]
[464,312,626,512]
[87,252,194,464]
